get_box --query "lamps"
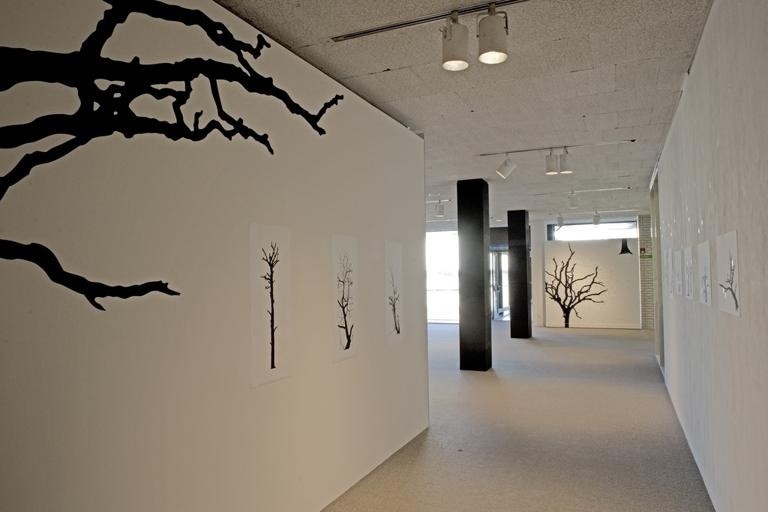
[329,1,530,72]
[425,198,451,218]
[479,144,574,177]
[557,210,638,227]
